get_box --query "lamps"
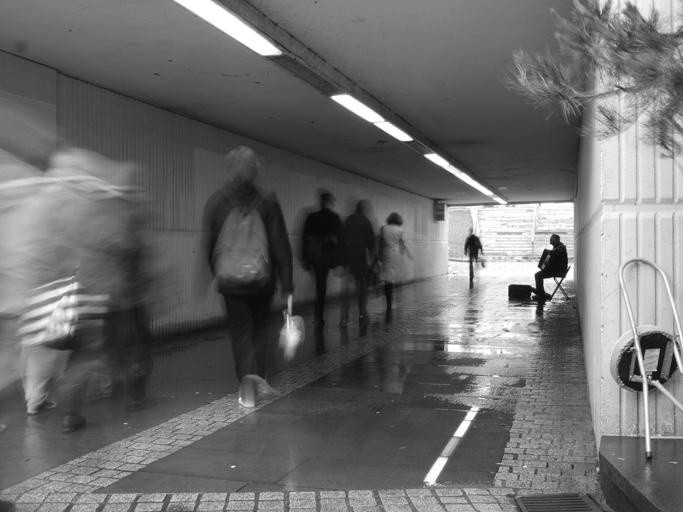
[169,0,285,57]
[330,88,509,205]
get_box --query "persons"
[338,197,376,327]
[296,189,342,327]
[465,227,482,287]
[199,144,296,410]
[532,233,568,301]
[372,211,413,322]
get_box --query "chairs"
[608,257,683,460]
[549,265,571,301]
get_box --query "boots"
[235,377,258,409]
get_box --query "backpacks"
[211,205,278,287]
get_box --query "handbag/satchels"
[15,275,116,350]
